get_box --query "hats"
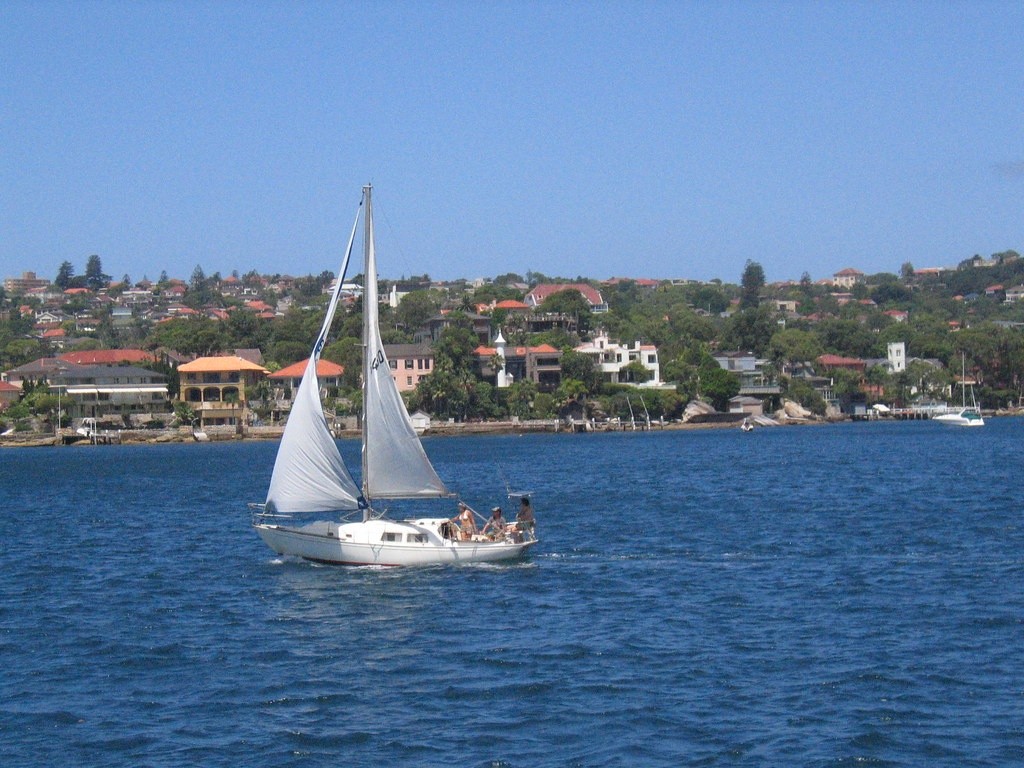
[458,503,465,507]
[491,507,501,512]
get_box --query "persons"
[450,503,478,541]
[510,497,534,544]
[480,506,507,543]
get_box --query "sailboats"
[931,351,985,426]
[248,181,540,567]
